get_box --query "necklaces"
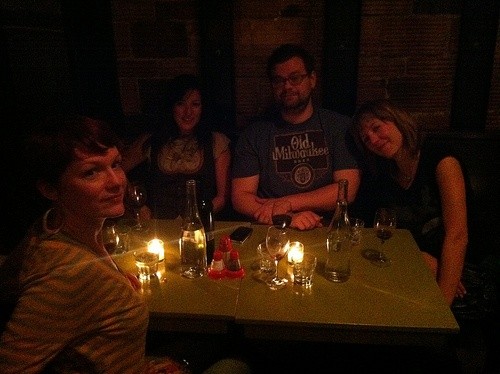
[405,174,412,181]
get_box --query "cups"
[102,223,128,254]
[272,201,293,235]
[287,242,304,264]
[291,253,318,286]
[133,249,161,281]
[350,219,365,247]
[147,238,164,262]
[256,242,276,275]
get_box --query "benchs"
[115,122,500,374]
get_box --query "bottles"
[198,199,214,265]
[179,180,207,279]
[323,179,351,283]
[209,235,240,272]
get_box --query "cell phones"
[230,226,254,244]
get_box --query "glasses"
[273,71,312,87]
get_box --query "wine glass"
[373,209,396,264]
[265,225,290,290]
[123,186,148,233]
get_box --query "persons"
[0,115,251,374]
[231,44,363,230]
[345,101,480,308]
[135,75,231,220]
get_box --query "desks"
[235,225,461,350]
[112,217,252,334]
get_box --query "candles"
[148,240,165,261]
[288,244,305,263]
